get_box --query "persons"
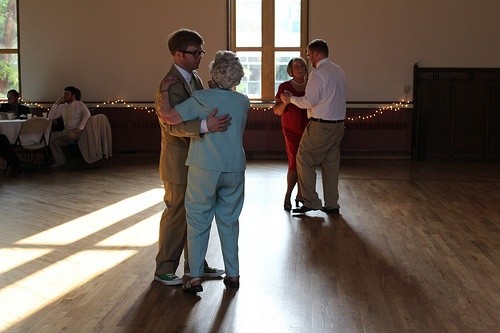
[0,90,31,162]
[48,86,90,169]
[154,29,250,294]
[273,38,346,215]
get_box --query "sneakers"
[154,273,183,284]
[184,267,224,277]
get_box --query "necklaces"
[292,78,307,86]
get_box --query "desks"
[0,119,52,162]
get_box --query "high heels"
[295,199,305,207]
[284,197,291,211]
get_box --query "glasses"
[178,50,206,59]
[306,51,319,58]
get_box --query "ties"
[191,73,196,93]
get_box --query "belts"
[310,117,345,123]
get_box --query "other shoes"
[51,162,66,168]
[320,207,339,213]
[34,154,42,168]
[224,276,239,286]
[9,161,22,175]
[188,277,201,285]
[293,204,319,213]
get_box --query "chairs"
[3,117,51,176]
[68,114,113,170]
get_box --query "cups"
[43,113,47,118]
[7,113,12,119]
[27,114,32,120]
